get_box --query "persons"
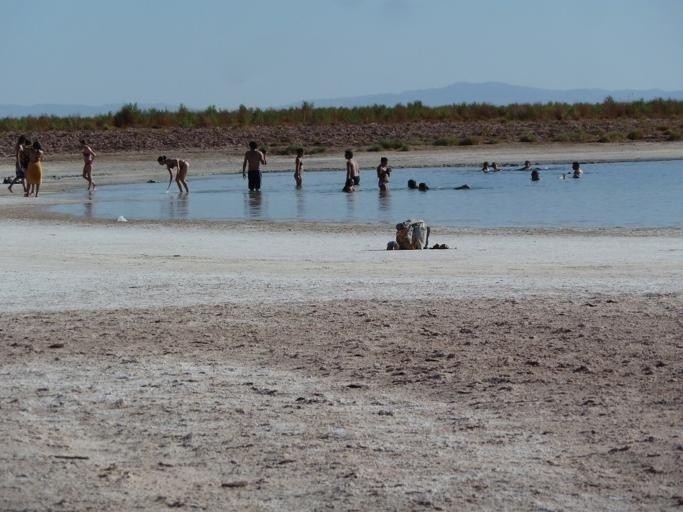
[22,141,44,198]
[80,139,96,191]
[571,161,583,179]
[418,182,428,192]
[292,147,303,190]
[407,179,418,189]
[21,140,46,194]
[156,155,189,194]
[492,162,500,172]
[5,134,26,193]
[395,222,417,249]
[342,177,356,192]
[452,183,471,190]
[241,141,265,191]
[518,160,532,171]
[376,157,392,192]
[481,161,489,172]
[344,150,360,185]
[530,170,539,181]
